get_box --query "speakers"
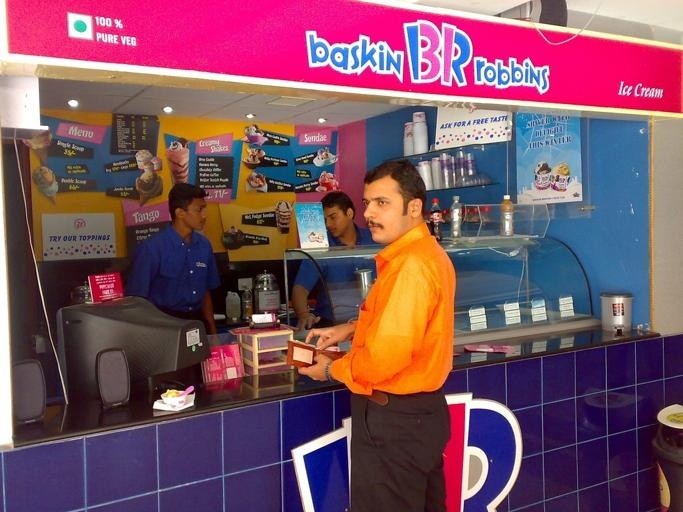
[12,359,46,425]
[96,347,131,411]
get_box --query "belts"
[366,389,390,407]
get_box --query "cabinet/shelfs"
[236,323,293,377]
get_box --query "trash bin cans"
[651,404,683,512]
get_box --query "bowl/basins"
[247,135,262,143]
[242,157,262,169]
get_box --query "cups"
[414,150,493,191]
[165,147,190,185]
[277,212,291,234]
[354,268,373,303]
[316,153,338,166]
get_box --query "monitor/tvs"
[55,296,210,400]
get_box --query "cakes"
[222,226,245,250]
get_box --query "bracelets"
[324,360,340,387]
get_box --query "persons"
[125,183,221,386]
[297,159,457,512]
[290,190,385,333]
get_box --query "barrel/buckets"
[226,291,241,320]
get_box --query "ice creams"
[275,200,293,234]
[318,172,338,193]
[242,149,265,163]
[550,164,570,192]
[533,160,552,190]
[242,124,269,146]
[22,131,189,207]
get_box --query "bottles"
[449,196,463,238]
[499,195,514,237]
[428,197,442,242]
[241,288,253,321]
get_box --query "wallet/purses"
[284,336,347,369]
